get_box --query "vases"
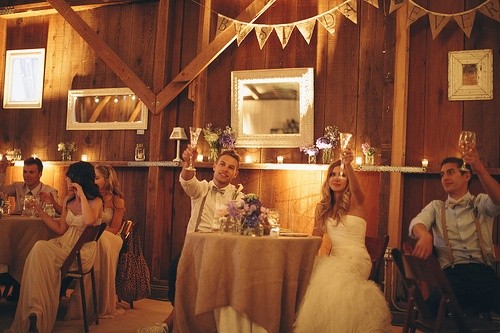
[322,149,334,163]
[62,151,71,160]
[365,155,376,166]
[208,142,219,162]
[308,156,317,164]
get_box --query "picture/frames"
[3,48,45,109]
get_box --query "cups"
[196,155,203,163]
[421,159,429,168]
[277,155,283,164]
[356,156,362,167]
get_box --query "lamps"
[169,127,188,161]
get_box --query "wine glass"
[457,131,476,171]
[266,207,279,230]
[183,127,202,171]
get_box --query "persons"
[295,149,391,333]
[139,143,244,333]
[0,157,58,215]
[8,162,104,333]
[409,144,500,333]
[64,165,126,319]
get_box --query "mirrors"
[231,67,313,148]
[66,87,148,130]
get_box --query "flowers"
[217,193,280,230]
[301,125,380,155]
[57,141,78,151]
[202,122,236,150]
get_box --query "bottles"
[13,149,22,161]
[3,201,12,217]
[23,195,55,218]
[134,144,145,161]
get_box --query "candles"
[422,159,428,167]
[277,156,283,163]
[356,157,362,164]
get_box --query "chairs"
[2,220,135,333]
[366,235,500,333]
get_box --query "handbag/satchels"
[115,222,152,301]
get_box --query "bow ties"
[212,186,225,194]
[448,197,467,210]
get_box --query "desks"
[173,232,323,333]
[0,215,61,285]
